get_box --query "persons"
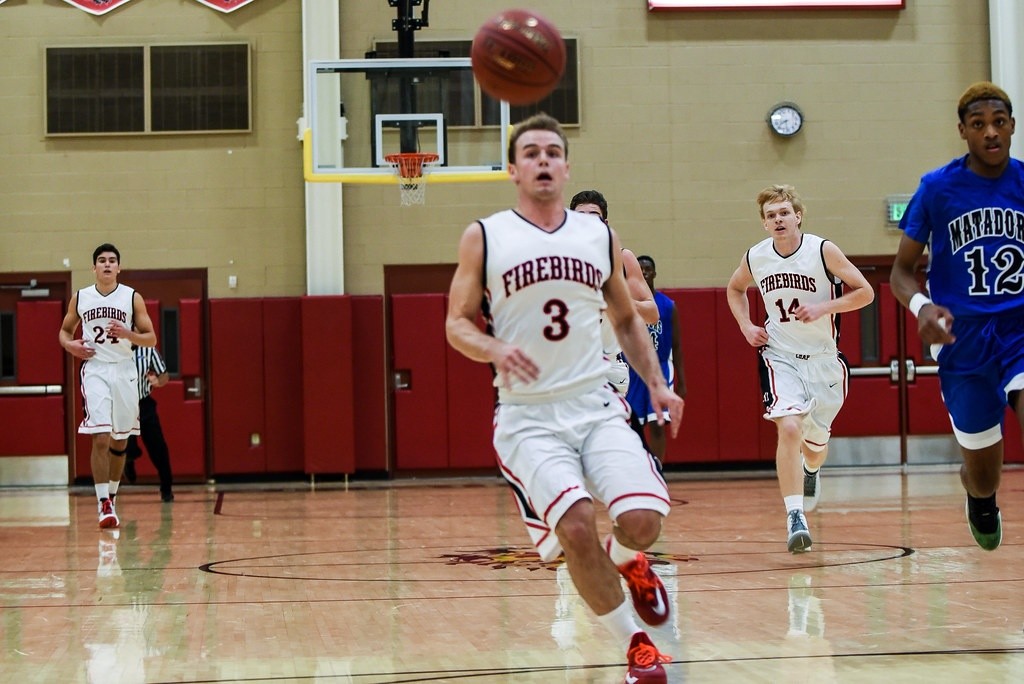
[570,187,661,428]
[726,183,875,554]
[446,113,686,684]
[126,324,176,503]
[617,255,677,465]
[889,80,1024,551]
[58,242,156,528]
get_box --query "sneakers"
[624,632,671,684]
[801,453,823,514]
[97,497,121,528]
[965,494,1007,548]
[786,507,815,553]
[604,535,670,626]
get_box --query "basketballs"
[471,10,566,105]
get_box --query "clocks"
[767,102,805,137]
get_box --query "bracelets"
[909,292,934,318]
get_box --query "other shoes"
[160,479,174,501]
[124,461,138,483]
